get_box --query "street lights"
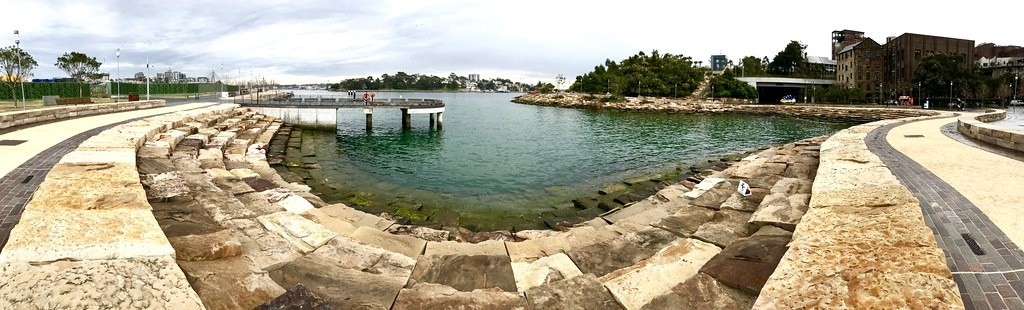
[918,82,922,105]
[1009,83,1012,107]
[1014,75,1018,113]
[13,29,27,110]
[115,46,122,103]
[950,81,953,110]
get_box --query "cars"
[947,98,968,107]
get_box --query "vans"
[1010,99,1024,106]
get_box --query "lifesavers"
[371,92,375,97]
[363,93,370,100]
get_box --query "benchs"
[55,98,95,107]
